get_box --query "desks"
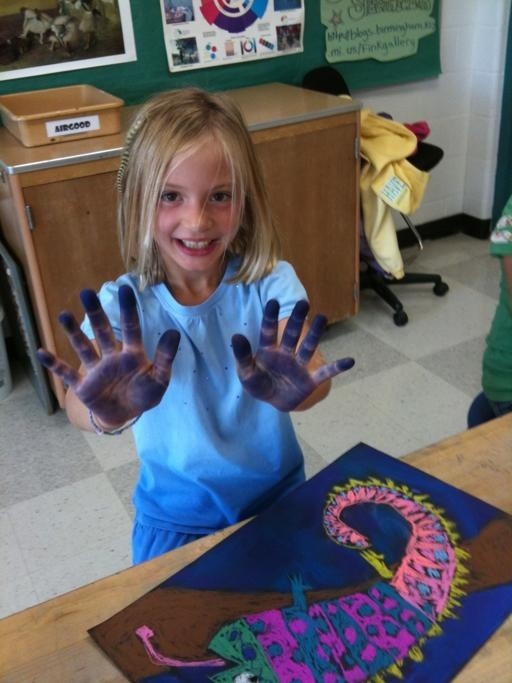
[0,411,512,683]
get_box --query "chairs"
[303,65,450,327]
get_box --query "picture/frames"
[0,0,137,81]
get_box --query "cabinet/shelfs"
[0,83,361,412]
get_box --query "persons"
[467,194,512,426]
[36,88,356,565]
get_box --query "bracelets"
[88,408,142,437]
